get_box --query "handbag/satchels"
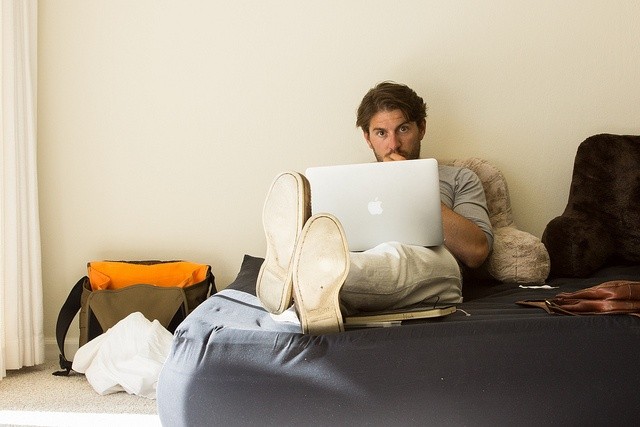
[53,257,217,378]
[515,280,640,320]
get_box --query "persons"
[256,83,493,337]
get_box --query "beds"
[157,252,639,425]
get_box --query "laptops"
[304,158,442,253]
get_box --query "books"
[344,303,456,324]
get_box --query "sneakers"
[292,210,351,336]
[256,166,312,317]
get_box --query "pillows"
[542,133,640,276]
[437,156,551,283]
